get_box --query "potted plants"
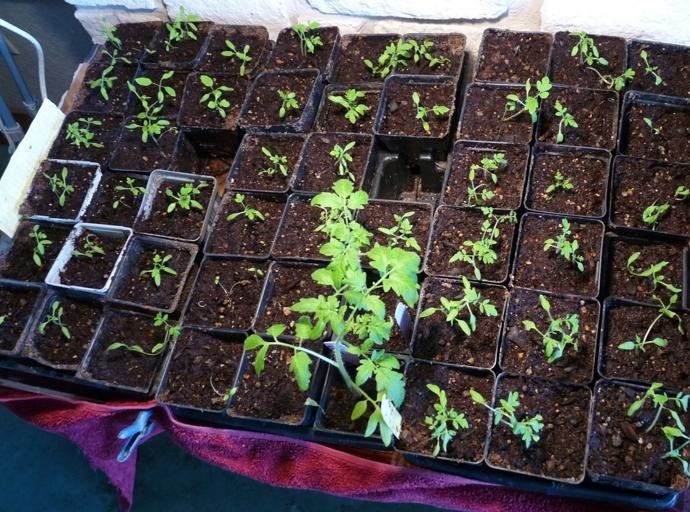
[2,14,689,507]
